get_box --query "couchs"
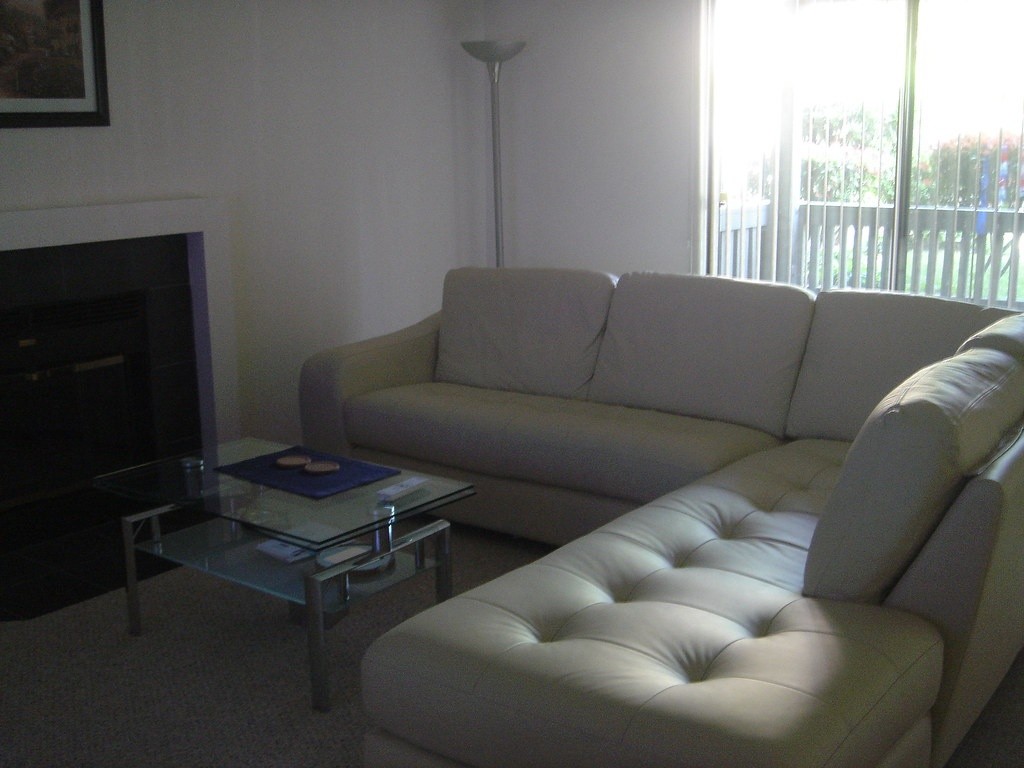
[299,268,1024,768]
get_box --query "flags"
[975,154,990,236]
[998,144,1009,207]
[1019,144,1024,198]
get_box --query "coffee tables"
[86,436,477,709]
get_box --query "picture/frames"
[0,0,112,128]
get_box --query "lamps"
[460,40,528,267]
